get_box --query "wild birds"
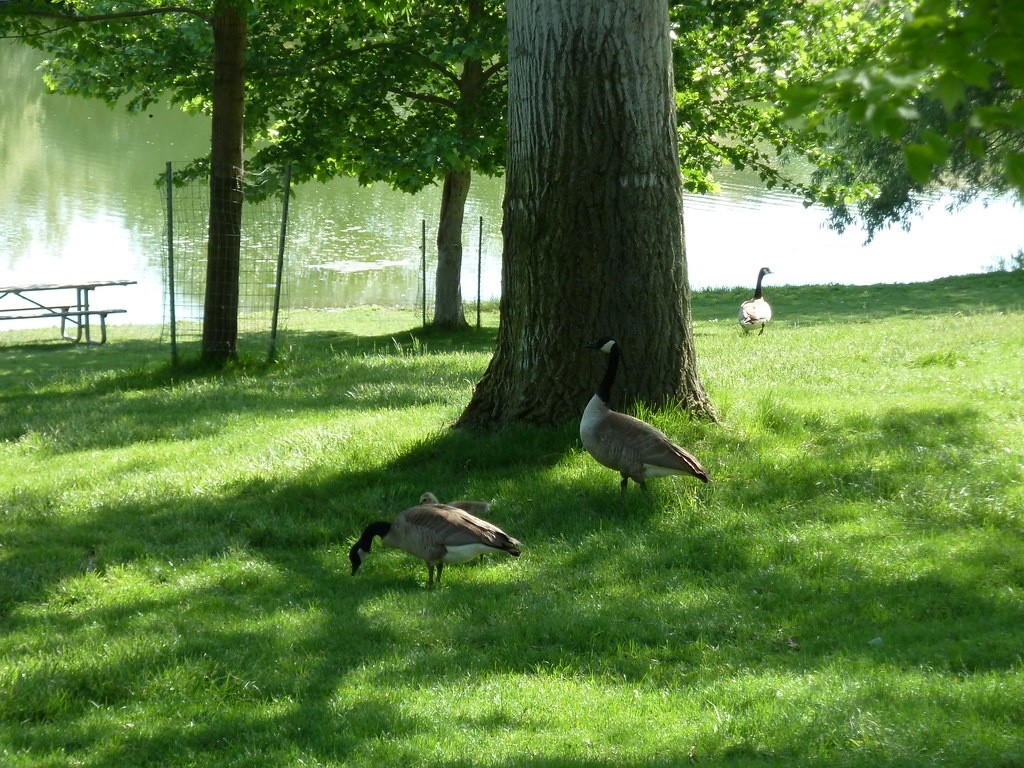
[738,267,775,335]
[580,340,709,491]
[348,492,522,581]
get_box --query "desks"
[0,280,138,343]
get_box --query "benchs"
[0,305,127,344]
